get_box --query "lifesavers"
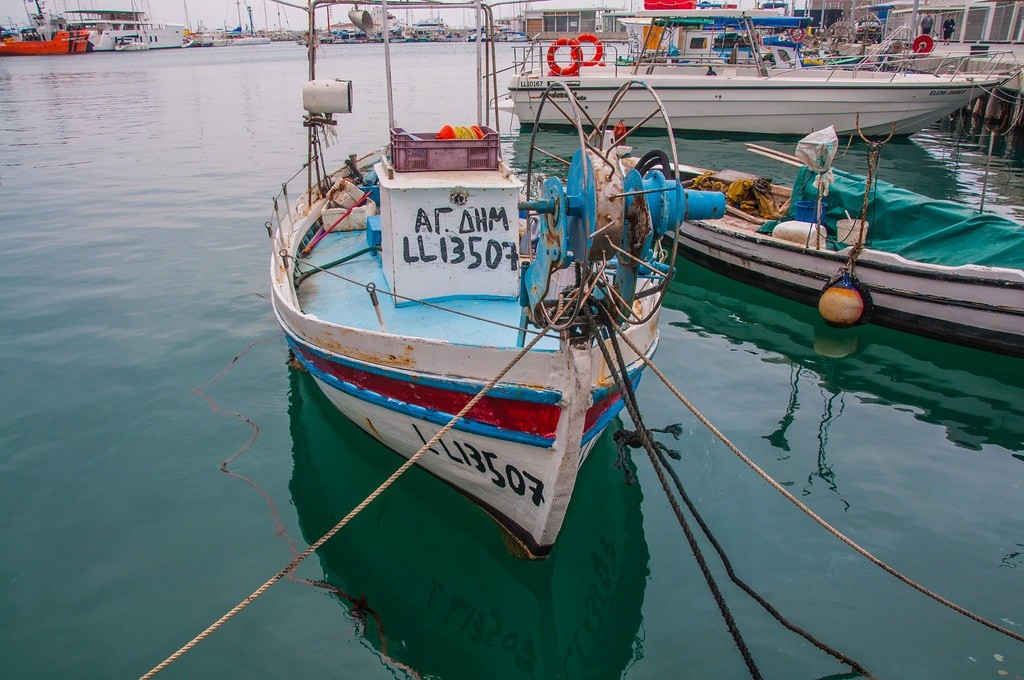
[790,27,805,43]
[570,34,603,66]
[547,38,583,77]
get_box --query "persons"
[943,14,956,46]
[920,12,934,35]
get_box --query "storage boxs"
[389,125,500,173]
[321,199,376,233]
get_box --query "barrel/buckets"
[837,218,869,246]
[794,201,828,226]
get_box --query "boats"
[284,344,649,679]
[263,0,728,564]
[614,155,1023,360]
[0,0,1023,142]
[655,243,1024,460]
